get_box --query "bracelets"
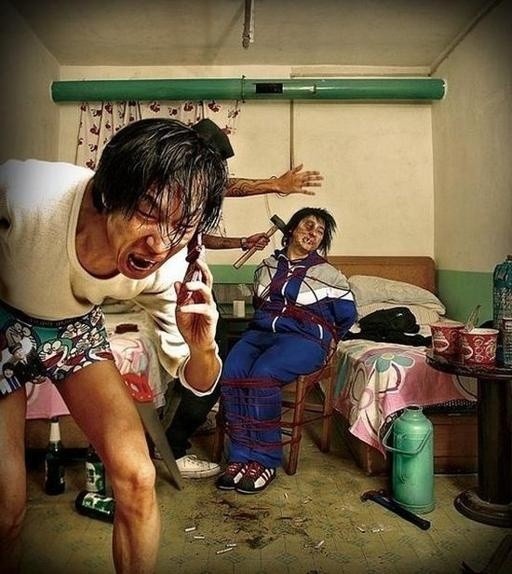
[238,235,249,251]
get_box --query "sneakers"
[193,418,216,435]
[175,454,276,494]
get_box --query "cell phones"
[174,222,208,307]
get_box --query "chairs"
[211,319,338,476]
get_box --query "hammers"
[360,490,430,530]
[233,214,286,270]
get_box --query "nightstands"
[218,303,256,360]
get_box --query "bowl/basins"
[427,320,499,365]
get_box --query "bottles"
[74,440,115,524]
[43,415,67,495]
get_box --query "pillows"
[346,272,448,317]
[100,296,136,314]
[356,301,441,325]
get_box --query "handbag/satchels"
[359,307,419,334]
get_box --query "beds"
[24,310,187,494]
[316,254,480,480]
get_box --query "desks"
[424,346,512,528]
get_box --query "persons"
[150,116,331,483]
[213,203,364,497]
[0,115,229,574]
